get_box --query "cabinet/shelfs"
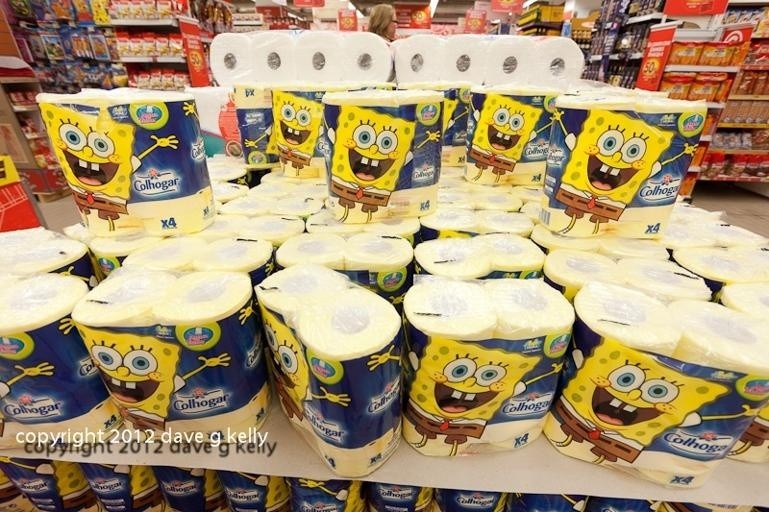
[0,0,313,96]
[486,0,769,184]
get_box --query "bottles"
[581,0,666,90]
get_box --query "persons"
[367,4,398,43]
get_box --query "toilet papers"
[0,28,768,512]
